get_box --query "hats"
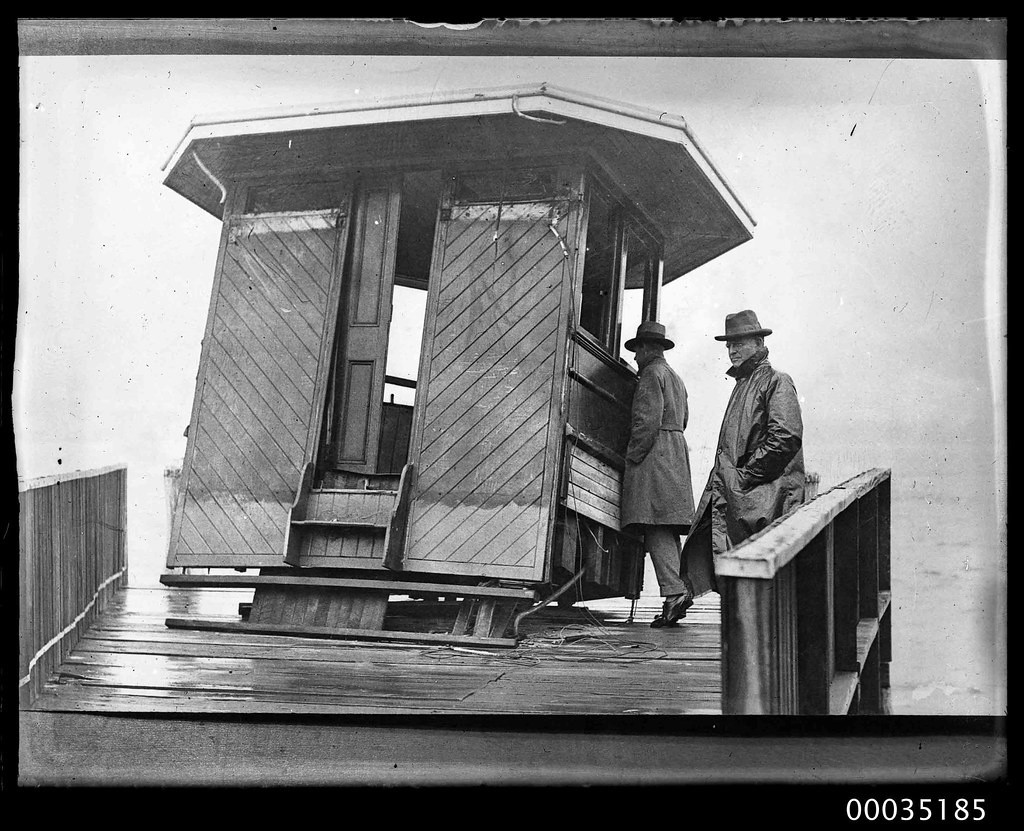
[714,309,773,341]
[624,321,674,352]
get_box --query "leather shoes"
[650,594,693,628]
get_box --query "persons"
[624,321,695,626]
[678,310,805,596]
